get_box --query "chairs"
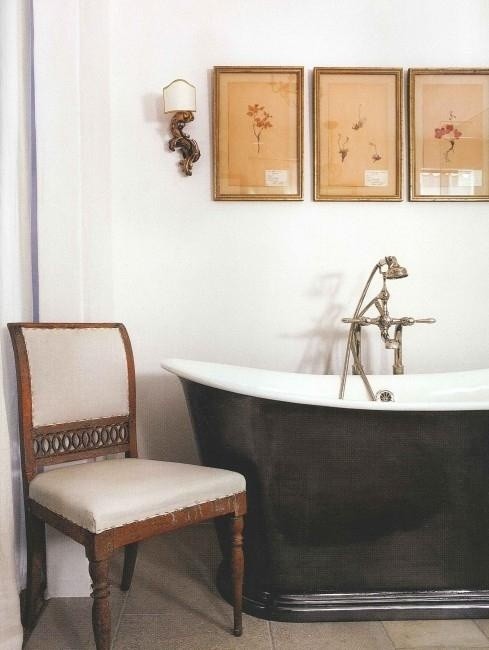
[6,323,249,650]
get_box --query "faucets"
[380,316,400,349]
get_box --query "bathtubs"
[162,356,489,620]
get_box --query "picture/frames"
[409,68,489,202]
[313,67,403,202]
[212,65,305,201]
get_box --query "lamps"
[163,79,200,176]
